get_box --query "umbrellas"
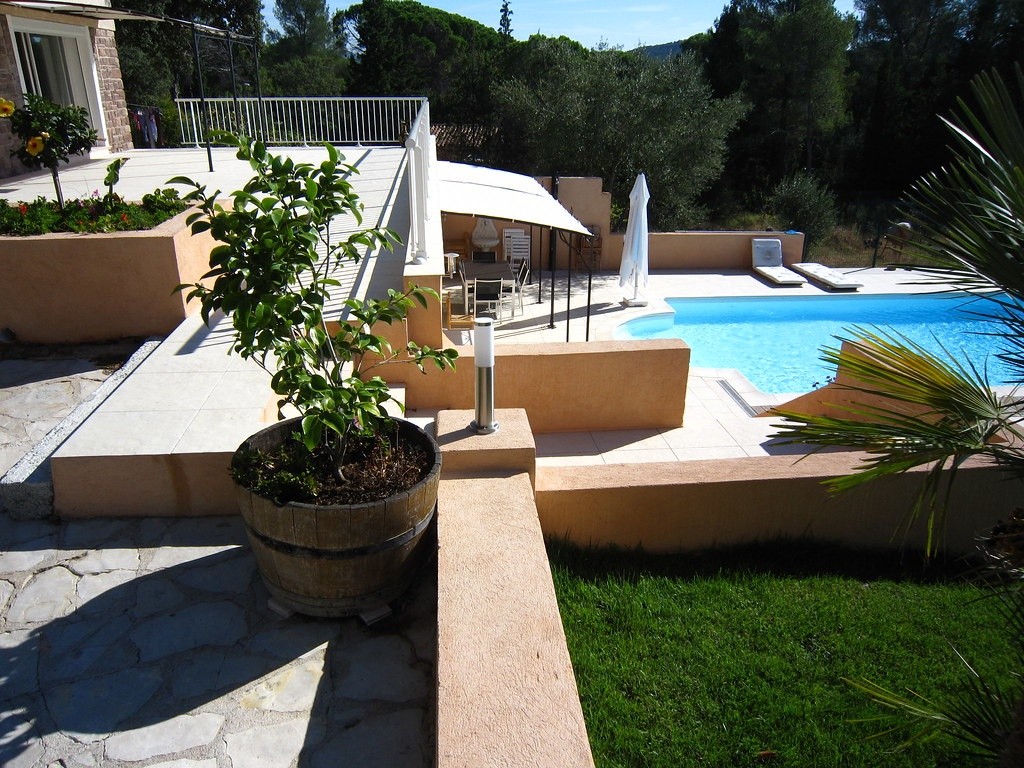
[619,170,651,287]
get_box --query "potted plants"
[161,124,462,624]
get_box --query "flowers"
[0,91,190,235]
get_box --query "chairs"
[471,250,498,261]
[458,259,465,273]
[790,261,864,292]
[458,268,476,298]
[502,255,526,308]
[500,264,531,316]
[446,292,475,329]
[471,277,505,325]
[751,237,809,285]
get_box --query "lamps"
[443,249,460,281]
[470,316,501,433]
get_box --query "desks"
[463,260,516,317]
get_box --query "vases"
[0,198,240,342]
